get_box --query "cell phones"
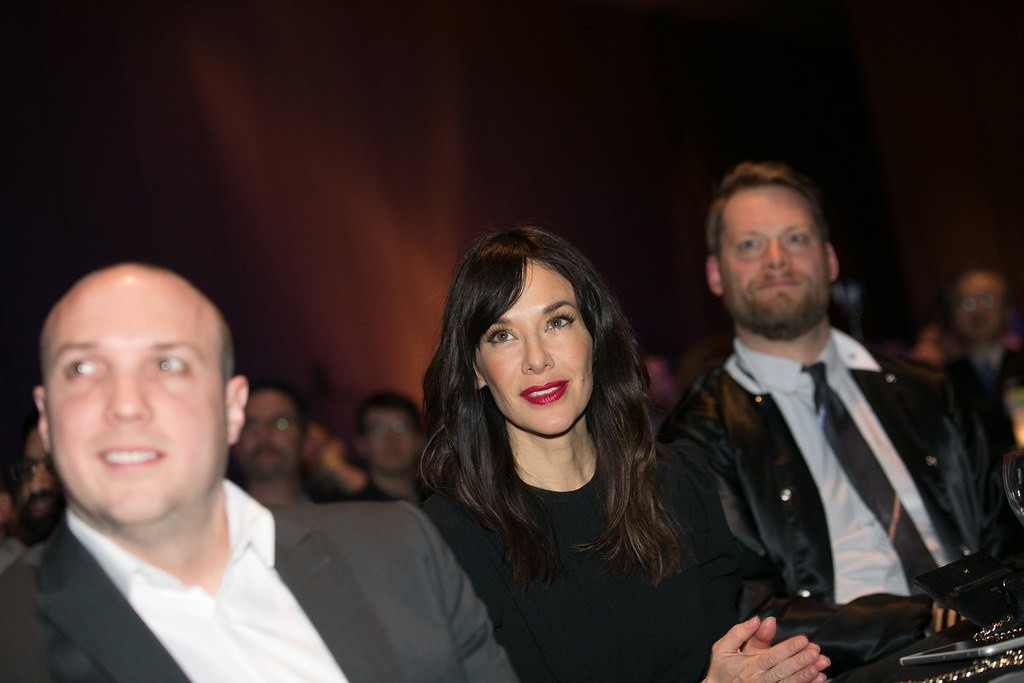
[900,635,1024,667]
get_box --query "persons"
[416,226,843,682]
[643,154,1022,682]
[1,264,522,683]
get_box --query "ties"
[802,361,940,598]
